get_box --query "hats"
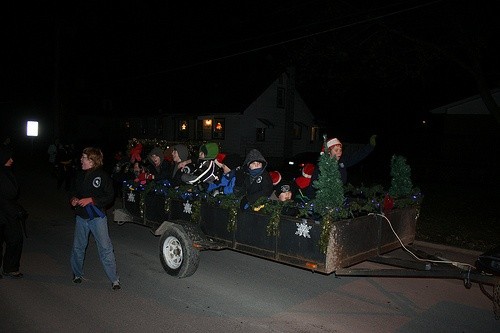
[302,163,314,178]
[270,171,281,185]
[320,138,343,155]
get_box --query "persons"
[208,158,241,195]
[233,148,274,212]
[152,146,188,192]
[63,144,79,190]
[0,134,11,151]
[303,137,346,220]
[0,147,28,280]
[276,163,321,224]
[67,146,121,290]
[180,143,222,192]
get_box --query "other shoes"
[3,271,24,278]
[112,279,120,288]
[0,274,3,279]
[72,275,81,284]
